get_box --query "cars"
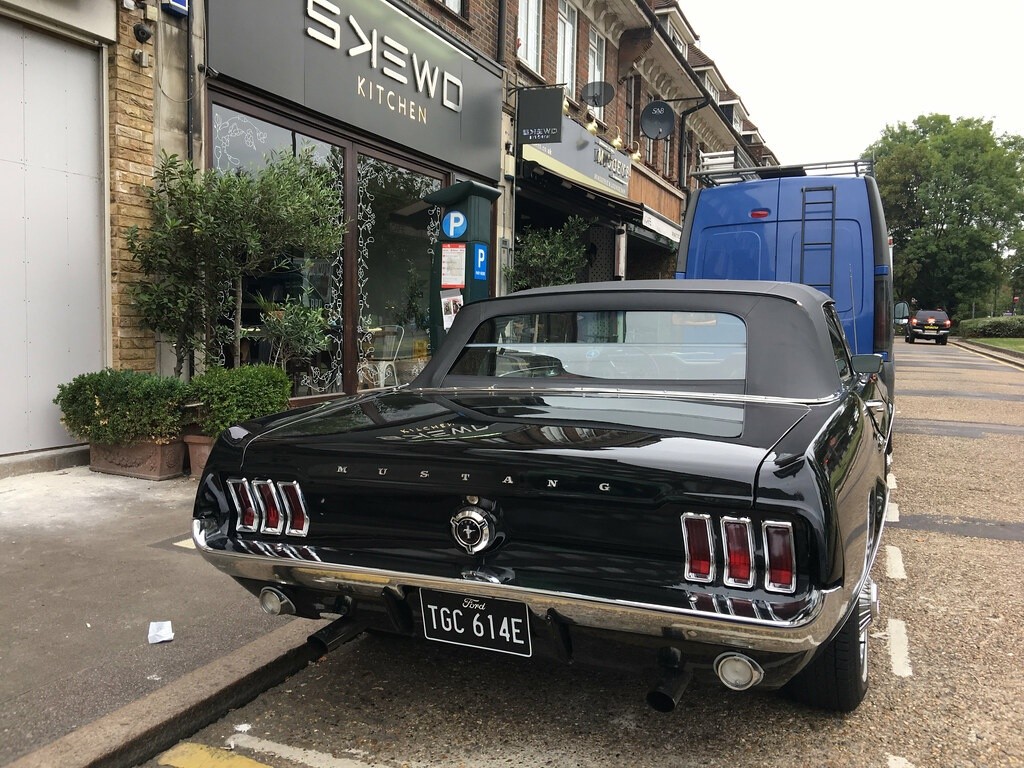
[1001,313,1016,317]
[191,279,897,714]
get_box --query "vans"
[656,148,911,456]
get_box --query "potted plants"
[182,362,296,481]
[51,366,184,482]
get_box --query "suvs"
[903,308,951,345]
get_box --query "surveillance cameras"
[133,24,152,42]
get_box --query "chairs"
[358,324,405,391]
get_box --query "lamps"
[569,108,599,134]
[620,141,645,163]
[598,124,624,149]
[561,86,571,113]
